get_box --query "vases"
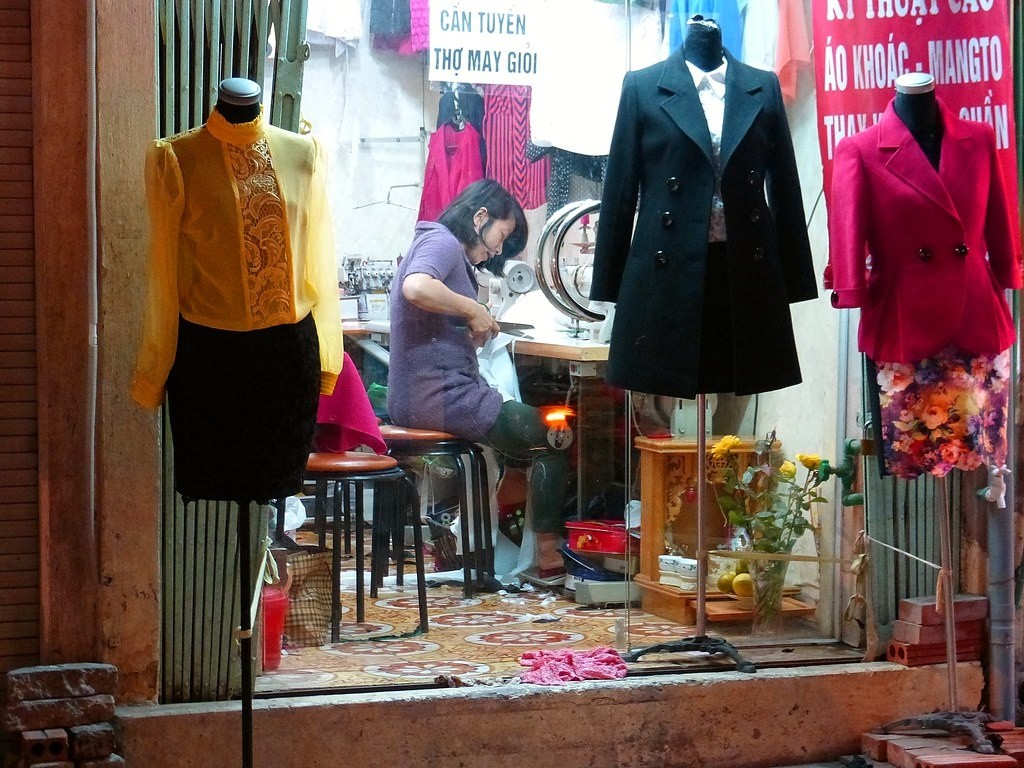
[745,535,794,639]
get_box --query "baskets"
[425,502,486,572]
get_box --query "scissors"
[491,319,535,339]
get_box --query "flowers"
[708,432,827,548]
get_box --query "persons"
[819,71,1024,481]
[384,178,573,581]
[131,78,346,510]
[586,12,822,405]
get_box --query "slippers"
[538,565,566,577]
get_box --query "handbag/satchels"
[282,543,333,648]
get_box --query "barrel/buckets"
[262,587,288,671]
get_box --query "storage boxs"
[564,519,638,555]
[264,588,286,672]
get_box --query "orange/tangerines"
[717,562,753,597]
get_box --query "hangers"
[428,82,476,149]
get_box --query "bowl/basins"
[675,558,697,576]
[658,555,682,571]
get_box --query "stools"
[301,452,429,643]
[378,424,492,600]
